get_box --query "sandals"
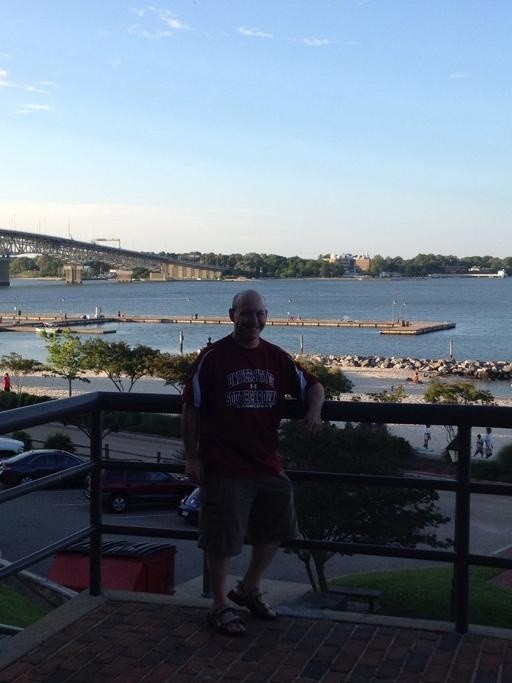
[227,579,277,621]
[207,606,247,637]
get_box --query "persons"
[411,372,419,384]
[482,428,496,459]
[178,288,327,638]
[2,371,13,393]
[421,424,432,449]
[472,433,485,457]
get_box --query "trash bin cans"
[50,539,175,596]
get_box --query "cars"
[0,437,91,491]
[83,460,201,523]
